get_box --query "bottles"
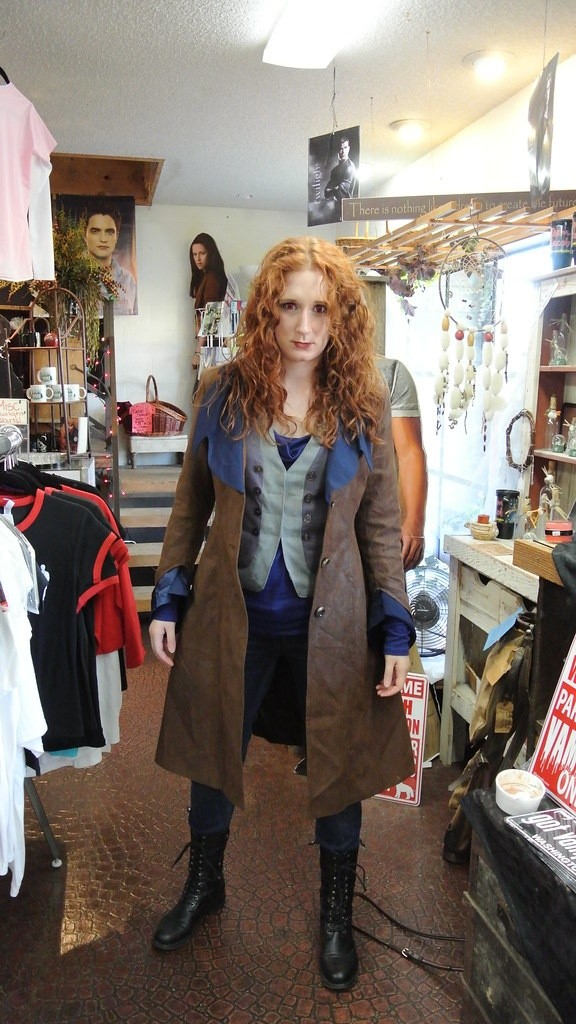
[544,394,576,457]
[43,330,64,347]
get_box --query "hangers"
[0,421,106,564]
[0,63,30,109]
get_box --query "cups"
[551,211,576,271]
[25,367,87,403]
[36,432,52,453]
[496,489,520,540]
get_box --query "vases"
[47,282,68,337]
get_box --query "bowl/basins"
[495,768,545,817]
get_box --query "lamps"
[388,119,430,150]
[461,48,506,88]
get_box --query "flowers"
[377,238,441,327]
[30,203,129,306]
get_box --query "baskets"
[125,371,186,437]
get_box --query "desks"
[438,531,547,769]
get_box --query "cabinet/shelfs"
[8,286,94,471]
[462,787,576,1024]
[516,264,576,539]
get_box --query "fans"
[402,563,450,656]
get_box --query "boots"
[318,846,368,989]
[151,828,226,951]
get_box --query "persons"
[189,231,233,412]
[71,202,138,314]
[324,136,357,218]
[148,231,428,991]
